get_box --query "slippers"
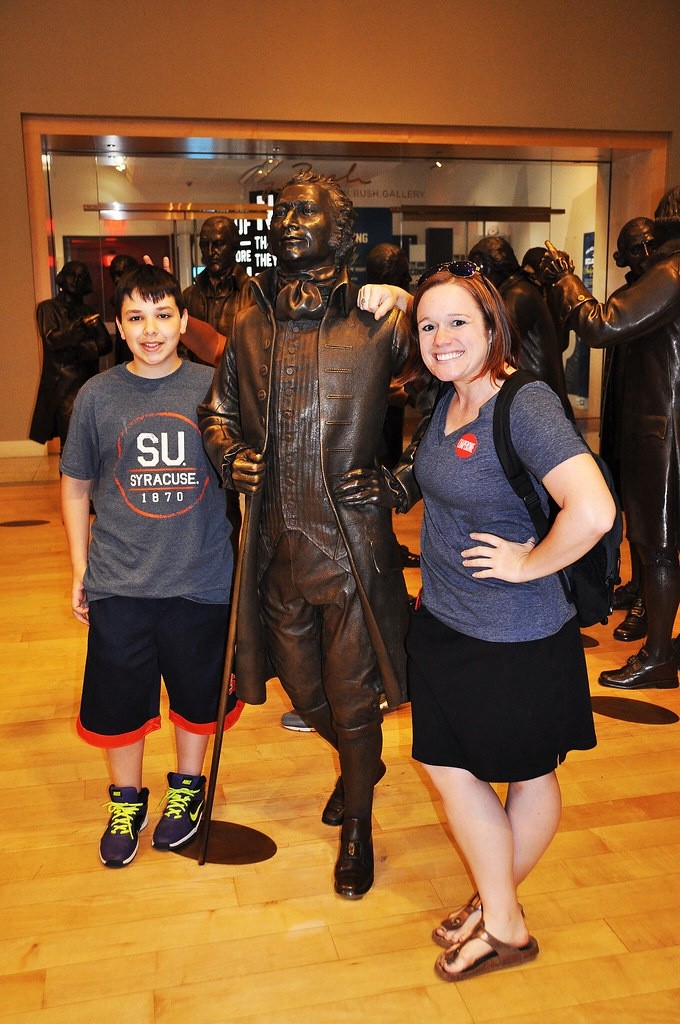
[431,891,525,948]
[435,918,539,982]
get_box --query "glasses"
[416,260,491,295]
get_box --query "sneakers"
[151,772,206,851]
[99,784,149,866]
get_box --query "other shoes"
[282,709,317,732]
[379,692,393,712]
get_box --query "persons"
[459,183,680,690]
[194,171,441,896]
[180,216,253,369]
[357,243,414,470]
[28,255,145,514]
[60,267,235,868]
[352,260,617,981]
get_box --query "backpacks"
[431,368,623,629]
